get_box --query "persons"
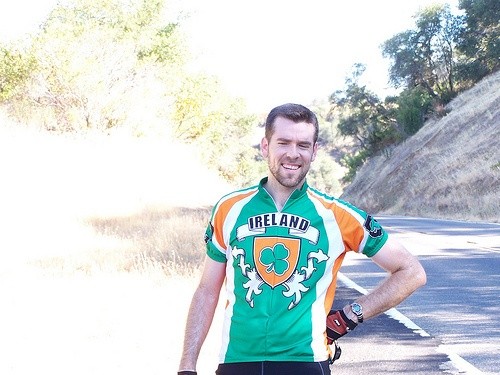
[177,103,428,375]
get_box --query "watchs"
[349,302,364,323]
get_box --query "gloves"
[178,371,197,375]
[326,309,358,345]
